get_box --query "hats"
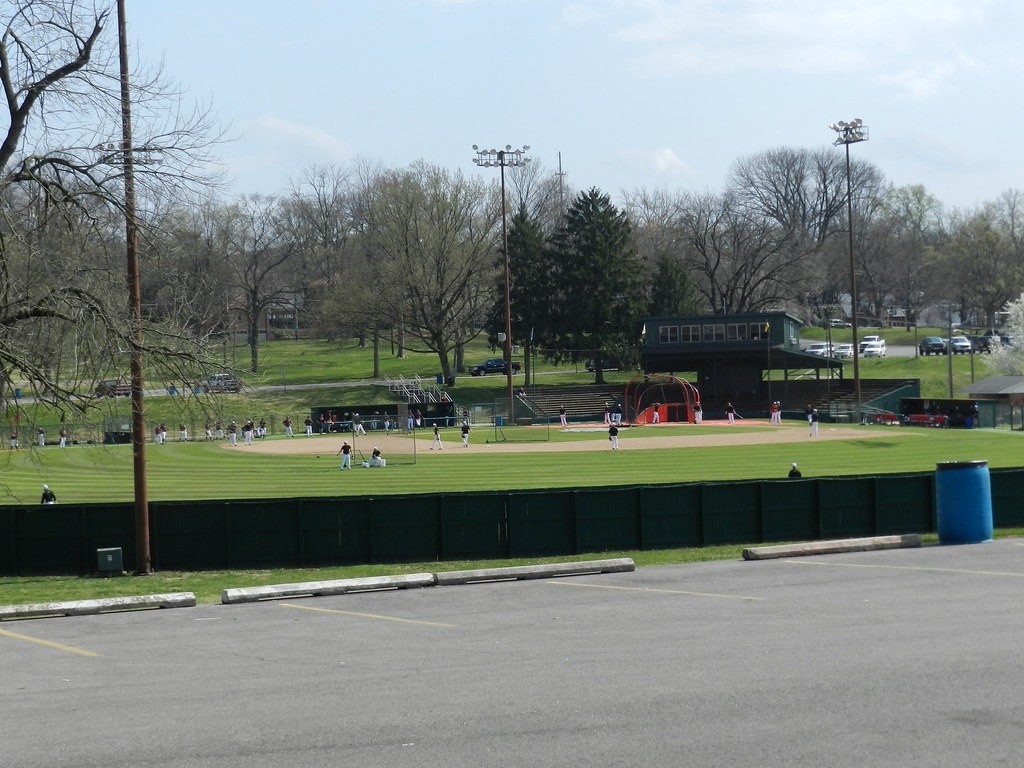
[44,485,49,490]
[344,441,348,444]
[374,447,378,450]
[232,421,235,423]
[247,421,250,424]
[433,423,436,426]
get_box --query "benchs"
[516,382,905,418]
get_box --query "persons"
[283,416,295,437]
[41,484,56,504]
[725,402,736,424]
[179,423,188,442]
[788,463,801,477]
[336,441,353,470]
[305,416,313,437]
[603,402,703,450]
[10,429,19,451]
[154,422,167,444]
[559,404,568,425]
[316,409,423,436]
[518,386,528,401]
[430,423,443,450]
[205,420,267,447]
[37,427,45,446]
[769,400,820,437]
[445,408,474,447]
[370,447,381,466]
[60,426,67,448]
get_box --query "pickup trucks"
[469,358,522,374]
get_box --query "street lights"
[472,142,535,424]
[830,119,868,429]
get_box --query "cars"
[860,336,880,354]
[830,318,852,330]
[863,340,885,359]
[808,343,830,358]
[834,345,857,359]
[971,337,995,353]
[946,336,971,353]
[919,335,948,355]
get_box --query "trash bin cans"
[495,416,502,426]
[437,375,443,384]
[964,416,974,428]
[168,386,176,396]
[934,460,994,546]
[15,389,22,399]
